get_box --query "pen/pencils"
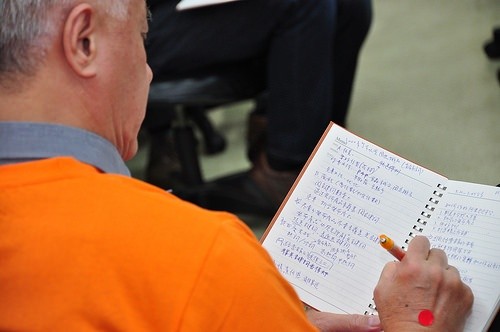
[379,235,406,261]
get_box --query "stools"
[141,67,274,219]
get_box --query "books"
[258,120,500,332]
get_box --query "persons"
[142,0,373,174]
[0,0,474,332]
